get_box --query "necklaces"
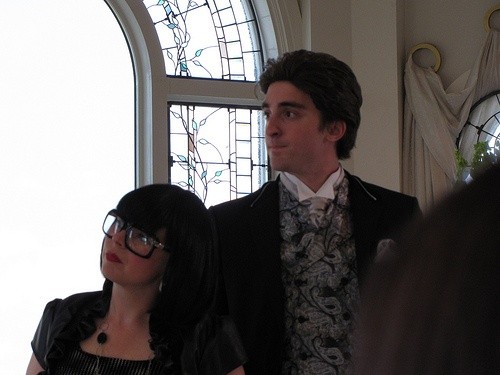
[95,318,155,375]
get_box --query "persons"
[25,182,247,375]
[157,47,424,375]
